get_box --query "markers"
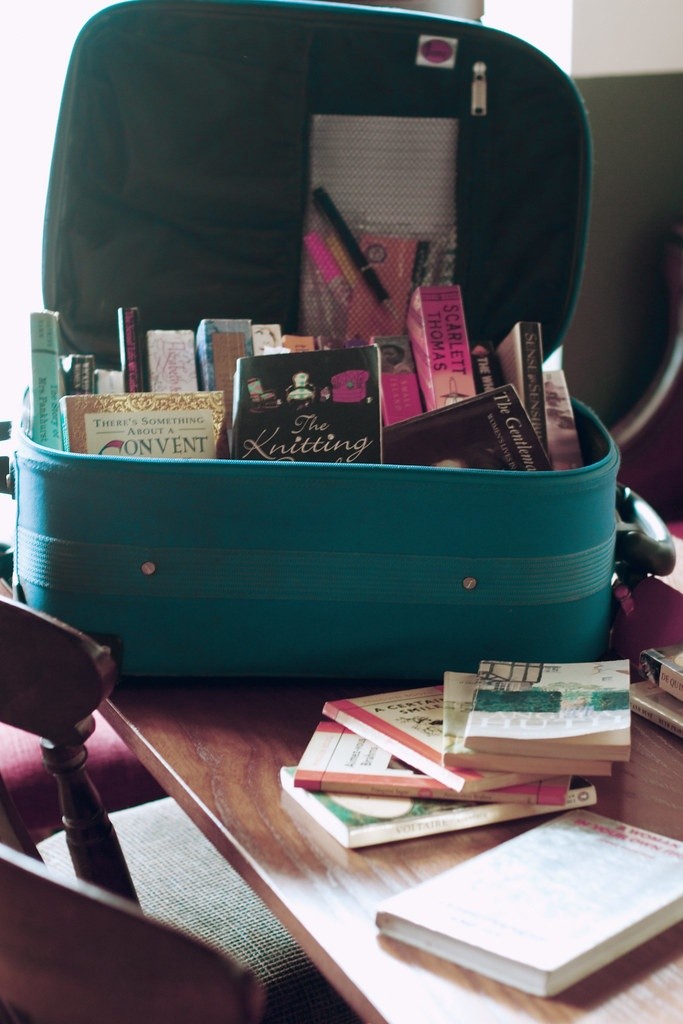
[304,233,352,315]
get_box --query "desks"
[99,630,683,1024]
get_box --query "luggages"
[6,0,676,679]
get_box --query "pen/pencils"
[313,186,403,326]
[325,233,389,328]
[412,224,457,290]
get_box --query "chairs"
[0,596,261,1024]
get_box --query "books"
[374,803,682,997]
[31,286,584,471]
[272,643,681,848]
